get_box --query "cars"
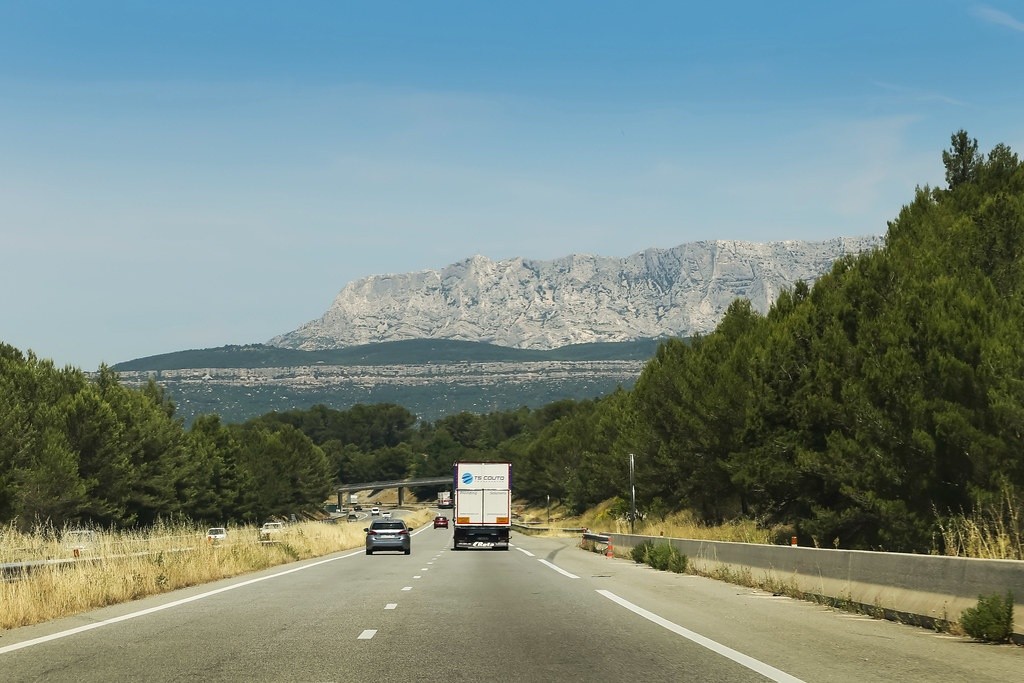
[363,519,412,555]
[260,522,284,539]
[346,513,359,523]
[206,528,228,544]
[433,517,449,529]
[354,505,362,512]
[382,511,393,518]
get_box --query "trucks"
[437,491,452,508]
[452,459,513,551]
[345,495,357,504]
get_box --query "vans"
[371,507,380,516]
